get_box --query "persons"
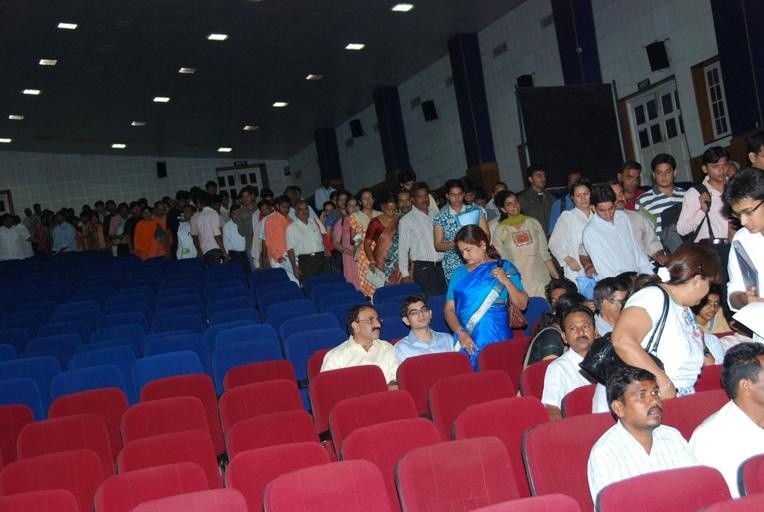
[0,180,332,292]
[689,344,764,498]
[587,367,693,509]
[320,133,764,416]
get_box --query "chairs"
[0,249,764,512]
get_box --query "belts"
[299,252,327,258]
[700,238,730,246]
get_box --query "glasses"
[731,201,764,218]
[360,318,383,324]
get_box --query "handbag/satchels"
[364,265,385,288]
[578,335,662,386]
[661,202,695,256]
